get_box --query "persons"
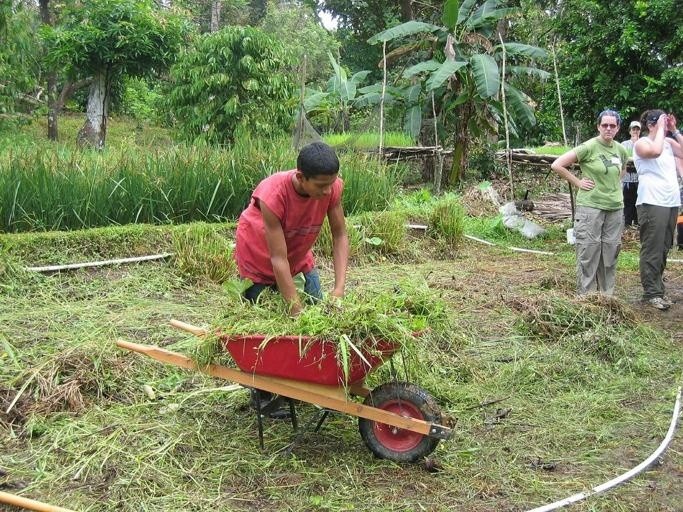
[551,110,683,309]
[235,141,348,418]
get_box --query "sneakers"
[642,295,677,310]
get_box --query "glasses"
[600,123,616,128]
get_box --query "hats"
[630,121,641,129]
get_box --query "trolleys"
[116,318,460,466]
